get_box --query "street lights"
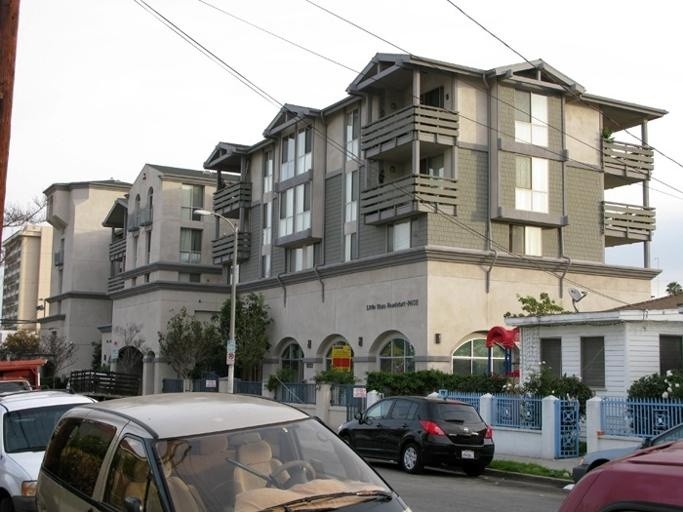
[193,209,240,395]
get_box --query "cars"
[556,424,682,512]
[37,392,409,511]
[1,380,33,395]
[338,396,495,475]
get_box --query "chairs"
[126,432,293,512]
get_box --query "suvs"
[1,387,99,512]
